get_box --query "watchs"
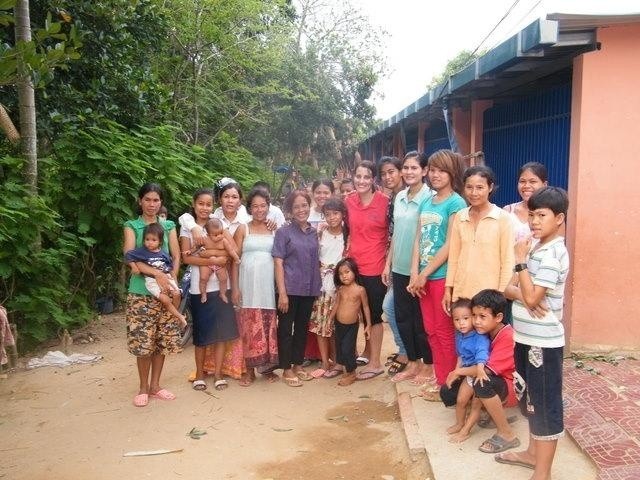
[514,263,527,272]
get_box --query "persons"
[123,183,181,407]
[308,198,347,379]
[381,155,408,376]
[124,222,187,329]
[324,258,371,387]
[503,161,565,244]
[339,178,355,198]
[158,205,167,218]
[231,188,279,387]
[306,177,344,229]
[494,186,571,480]
[177,188,241,391]
[182,217,242,305]
[406,149,465,403]
[252,180,287,235]
[446,288,526,453]
[441,165,517,324]
[382,151,435,387]
[217,183,277,241]
[213,176,247,224]
[444,297,491,444]
[271,189,323,387]
[341,160,391,380]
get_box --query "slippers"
[262,372,280,384]
[282,373,303,387]
[385,354,398,366]
[356,370,384,380]
[415,385,434,396]
[495,451,536,470]
[133,394,149,406]
[214,377,228,390]
[392,372,415,382]
[479,435,521,453]
[324,368,344,377]
[150,389,176,402]
[191,378,207,391]
[236,374,252,387]
[388,359,407,375]
[294,368,313,382]
[423,393,442,402]
[311,367,325,379]
[338,374,356,386]
[478,415,519,428]
[410,376,432,386]
[355,353,368,364]
